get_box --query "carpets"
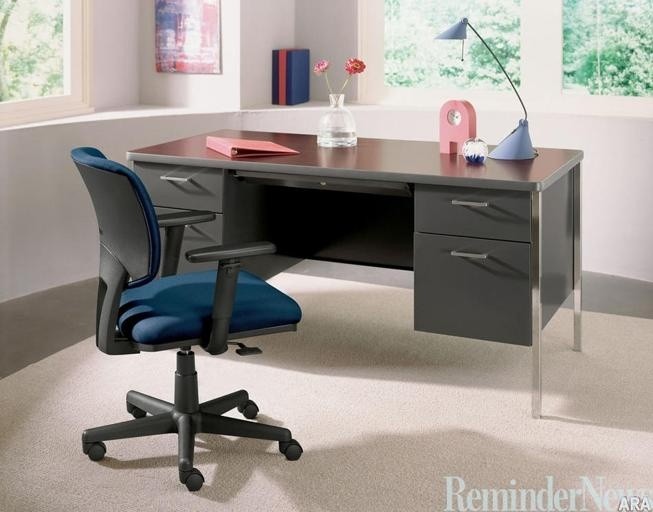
[2,258,652,512]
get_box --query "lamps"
[432,17,539,161]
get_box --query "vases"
[316,93,358,147]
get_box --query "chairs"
[68,144,303,493]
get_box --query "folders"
[206,136,300,159]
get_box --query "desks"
[125,127,585,421]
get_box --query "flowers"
[313,57,367,106]
